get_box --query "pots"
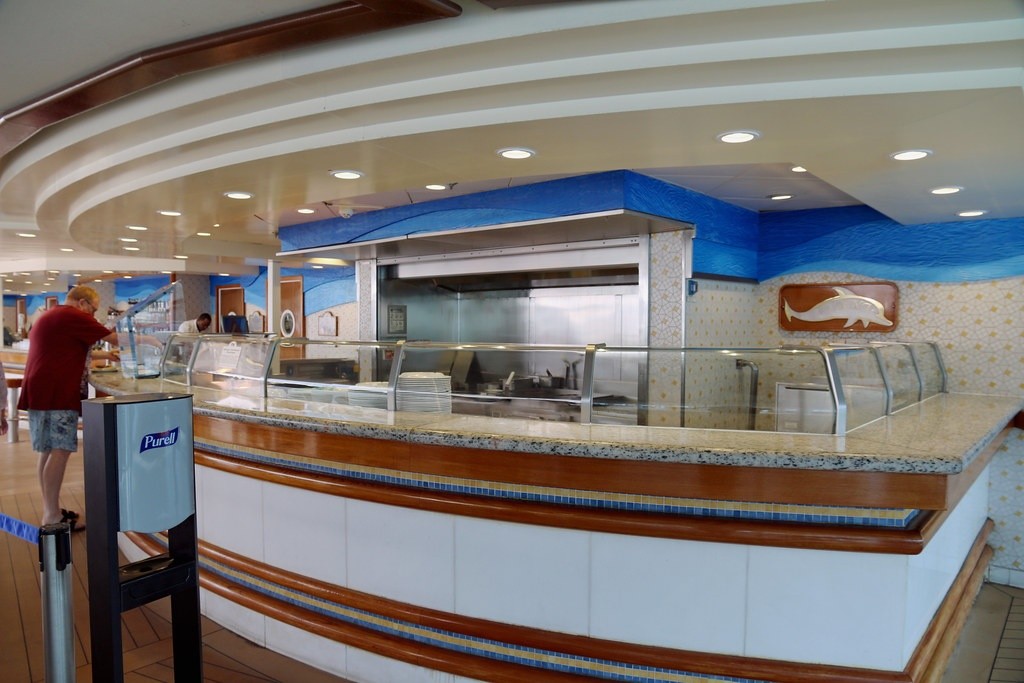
[539,376,566,389]
[498,378,533,389]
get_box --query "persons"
[17,287,162,532]
[0,361,9,436]
[178,313,211,355]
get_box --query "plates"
[397,372,451,413]
[288,388,387,409]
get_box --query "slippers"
[61,508,80,519]
[59,515,86,533]
